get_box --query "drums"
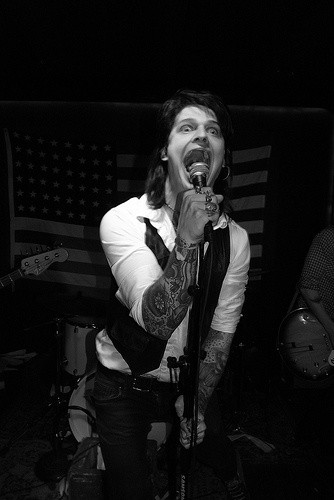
[62,293,111,377]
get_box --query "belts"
[97,361,176,392]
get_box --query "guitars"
[277,306,334,382]
[0,243,70,291]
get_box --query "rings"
[205,195,212,202]
[195,186,202,194]
[211,202,217,212]
[204,189,211,196]
[204,203,211,211]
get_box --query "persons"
[89,89,250,500]
[295,223,334,348]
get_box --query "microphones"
[190,161,214,243]
